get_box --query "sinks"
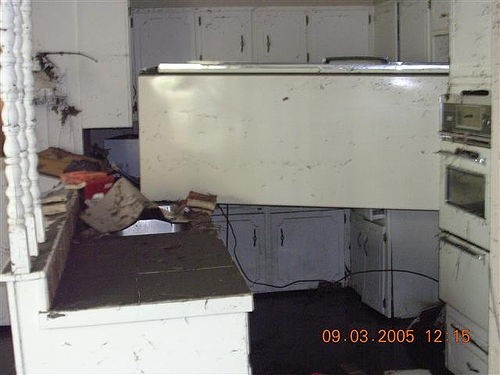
[110,204,191,235]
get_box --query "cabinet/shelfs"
[211,209,344,293]
[349,208,443,318]
[129,0,450,124]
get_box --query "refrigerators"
[136,54,450,210]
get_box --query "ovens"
[432,78,492,375]
